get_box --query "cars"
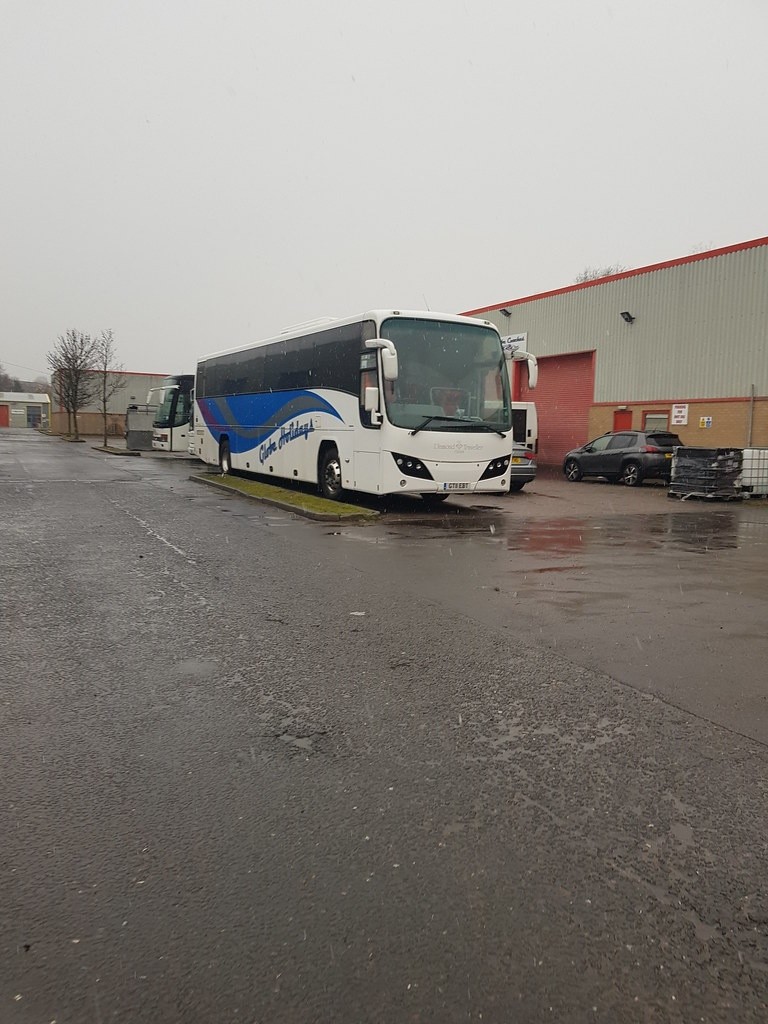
[509,441,537,493]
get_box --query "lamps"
[620,312,635,322]
[498,309,511,318]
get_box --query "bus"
[146,375,194,451]
[195,309,538,502]
[189,388,195,456]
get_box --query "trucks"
[483,401,539,456]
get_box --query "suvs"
[562,429,685,487]
